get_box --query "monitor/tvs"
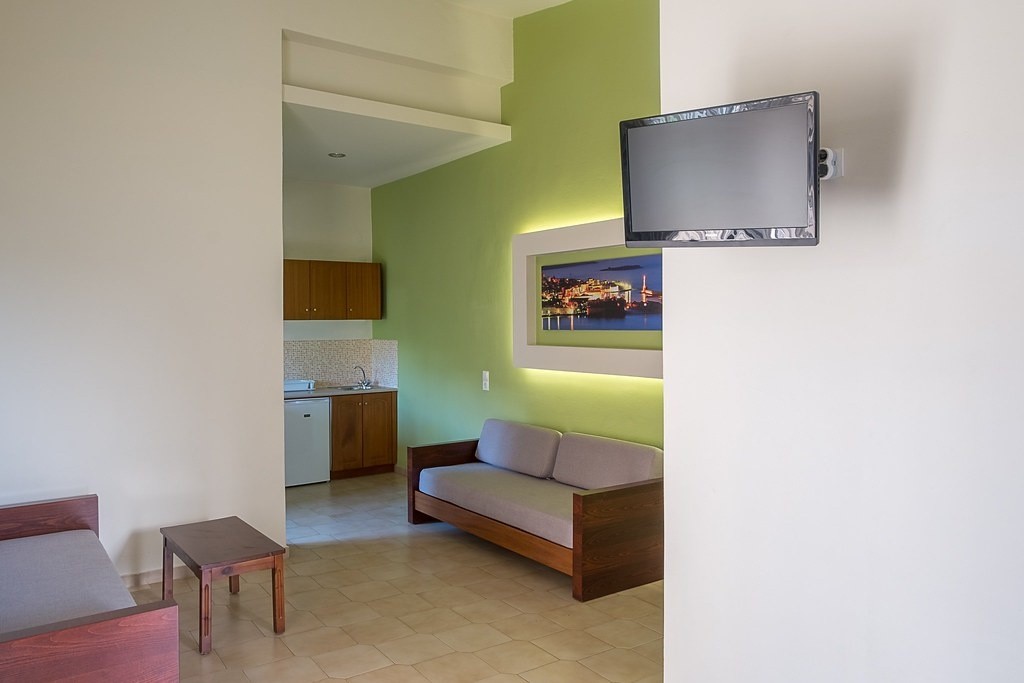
[619,91,821,249]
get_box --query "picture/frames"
[512,216,663,379]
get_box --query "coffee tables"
[160,515,287,654]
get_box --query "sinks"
[327,385,385,391]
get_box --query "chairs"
[0,494,180,683]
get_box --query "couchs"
[406,418,664,602]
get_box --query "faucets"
[354,365,372,387]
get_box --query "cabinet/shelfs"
[284,258,382,320]
[331,391,397,480]
